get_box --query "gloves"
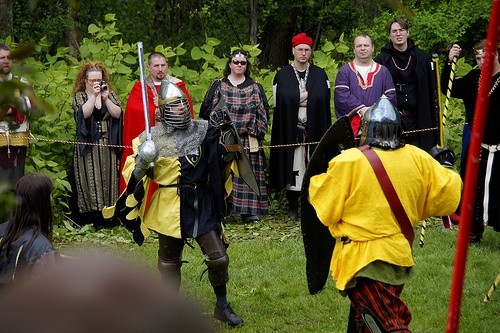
[136,133,159,162]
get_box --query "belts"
[481,144,500,223]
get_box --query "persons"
[269,32,332,220]
[0,42,36,195]
[120,51,195,195]
[306,91,463,332]
[102,75,246,328]
[66,61,124,230]
[199,48,271,222]
[333,31,398,147]
[373,16,441,155]
[0,173,59,289]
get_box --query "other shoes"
[287,207,299,221]
[242,212,261,223]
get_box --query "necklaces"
[477,72,500,97]
[391,54,412,70]
[290,61,312,92]
[440,39,500,242]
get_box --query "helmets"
[358,93,403,150]
[157,78,191,130]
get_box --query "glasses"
[232,60,248,65]
[85,76,102,83]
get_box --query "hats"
[292,33,313,48]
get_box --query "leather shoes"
[214,302,244,327]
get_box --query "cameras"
[99,80,107,90]
